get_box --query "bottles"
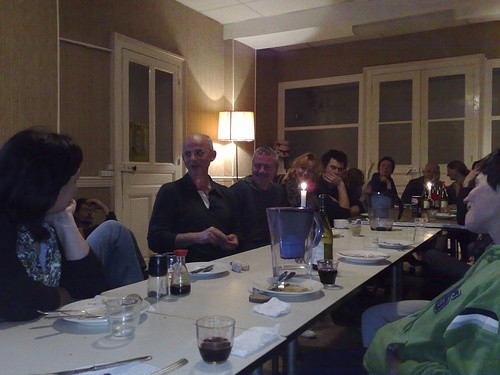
[311,194,333,271]
[170,250,191,295]
[147,256,169,298]
[422,185,449,210]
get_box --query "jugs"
[365,192,396,232]
[266,207,322,278]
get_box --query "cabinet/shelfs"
[277,52,500,198]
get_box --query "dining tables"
[1,206,458,375]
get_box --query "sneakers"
[300,329,316,338]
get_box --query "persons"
[277,151,323,232]
[456,160,491,226]
[73,197,149,280]
[343,168,374,215]
[446,160,475,203]
[399,160,447,212]
[146,133,249,262]
[319,149,350,228]
[362,150,500,375]
[0,130,112,322]
[228,146,316,338]
[363,157,423,266]
[420,233,494,298]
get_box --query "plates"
[337,250,390,264]
[185,262,228,277]
[378,242,409,249]
[58,299,150,326]
[252,277,324,298]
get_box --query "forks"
[276,272,295,291]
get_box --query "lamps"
[216,110,255,183]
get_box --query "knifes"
[191,265,214,273]
[267,271,287,290]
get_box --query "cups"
[317,259,339,287]
[334,219,349,229]
[106,298,138,337]
[414,218,426,234]
[196,315,235,364]
